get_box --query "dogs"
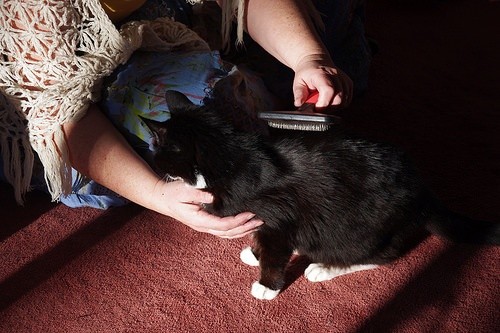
[138,90,498,295]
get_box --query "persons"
[0,0,353,238]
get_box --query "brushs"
[259,91,340,131]
